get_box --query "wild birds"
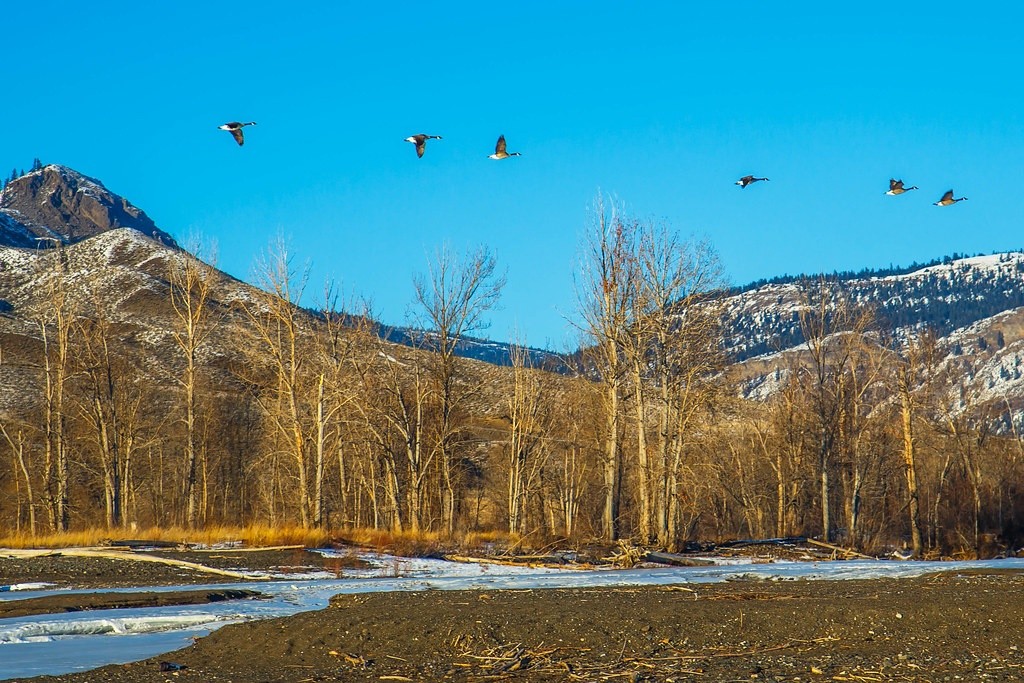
[734,175,770,190]
[487,134,522,160]
[404,134,442,158]
[218,122,256,146]
[933,189,968,206]
[884,178,919,195]
